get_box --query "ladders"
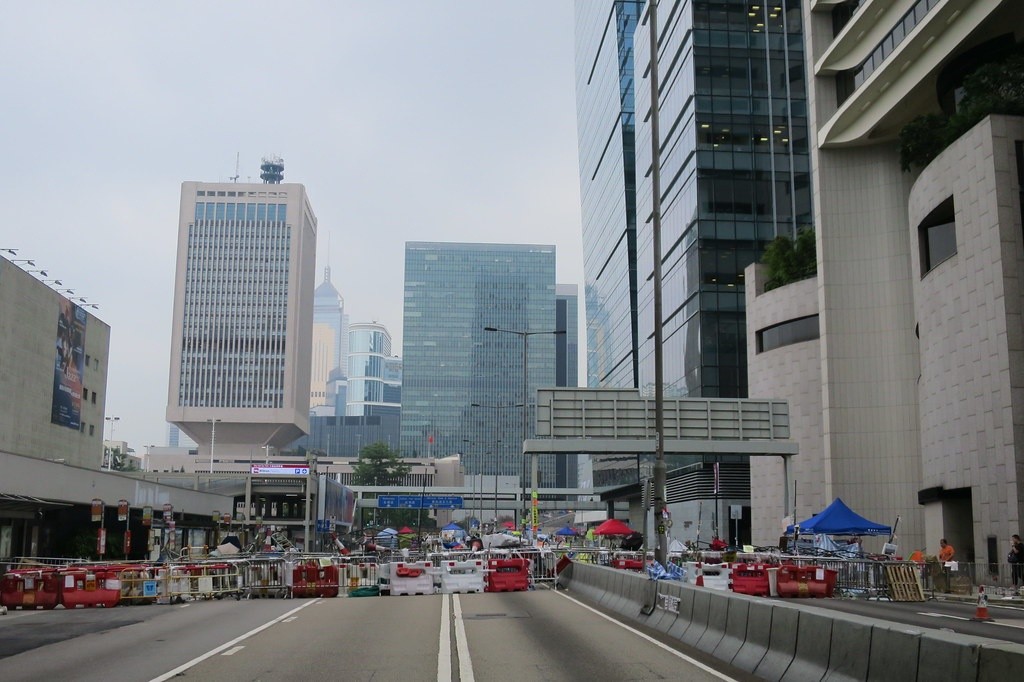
[271,532,298,552]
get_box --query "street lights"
[471,404,524,534]
[144,445,155,472]
[261,445,274,464]
[462,439,501,534]
[207,416,221,473]
[458,452,492,519]
[105,415,120,470]
[484,327,566,538]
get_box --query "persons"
[939,538,955,593]
[1008,534,1024,589]
[711,538,727,550]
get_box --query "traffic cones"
[331,533,349,555]
[968,586,996,622]
[364,541,390,552]
[696,561,704,587]
[469,539,484,552]
[265,528,272,552]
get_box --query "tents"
[376,526,415,536]
[592,518,634,547]
[442,523,465,535]
[556,524,578,541]
[786,498,891,540]
